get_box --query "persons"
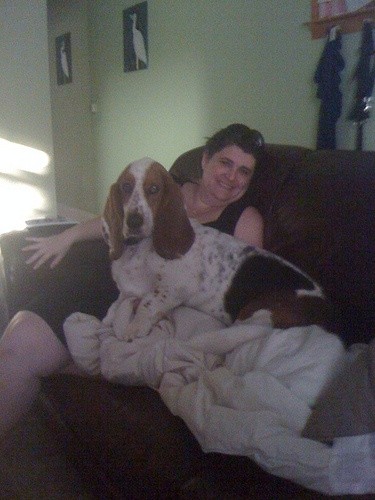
[1,122,265,444]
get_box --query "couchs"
[0,144,374,500]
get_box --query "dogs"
[99,157,337,343]
